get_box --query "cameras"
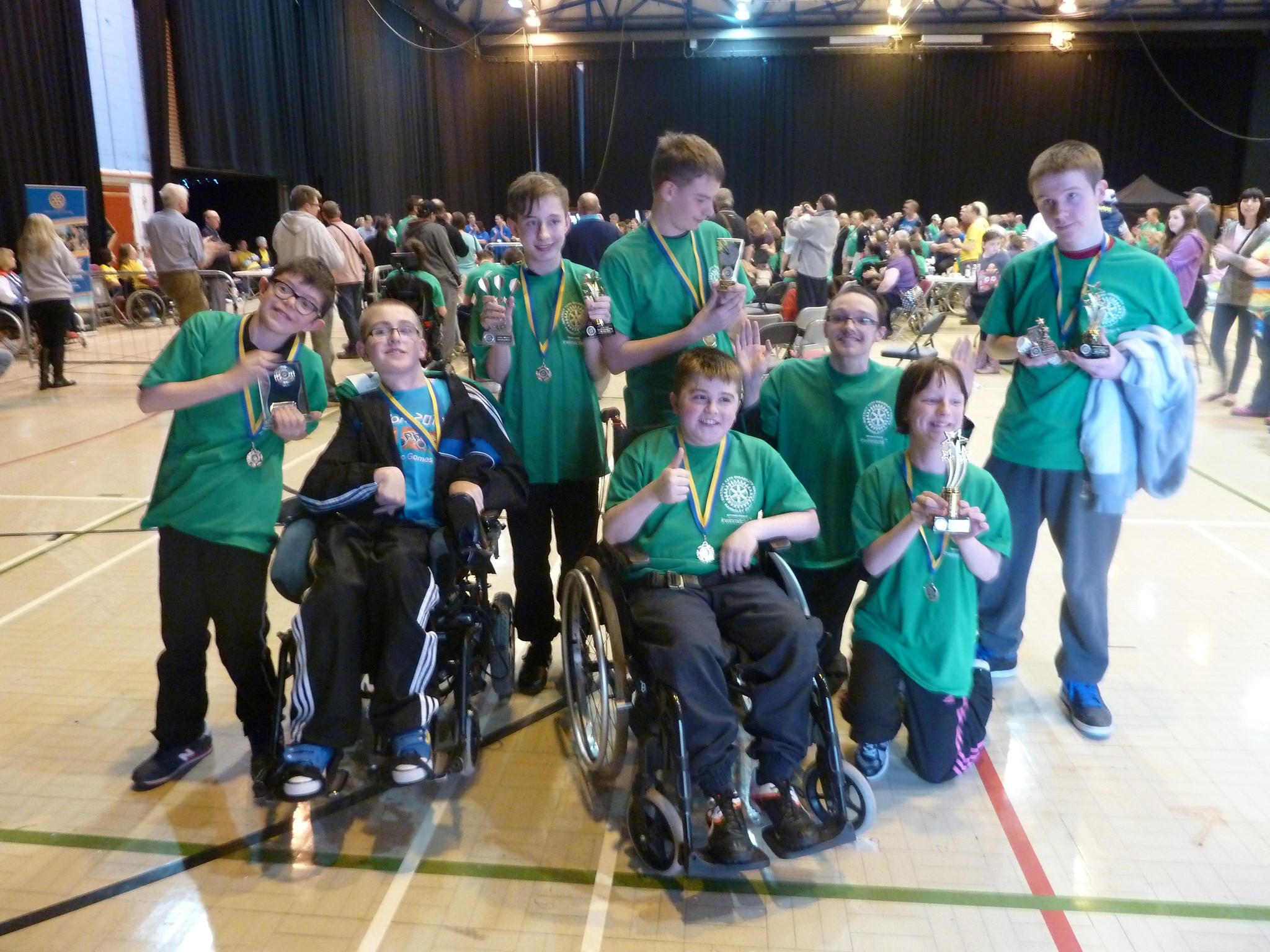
[256,359,313,428]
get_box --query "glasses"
[310,202,322,209]
[364,326,421,343]
[267,276,323,318]
[825,313,880,328]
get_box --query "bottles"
[965,264,971,278]
[953,262,959,273]
[972,263,976,278]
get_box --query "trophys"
[255,356,316,429]
[932,428,971,535]
[474,273,521,346]
[1025,317,1059,359]
[579,269,615,340]
[714,237,745,293]
[1074,281,1111,359]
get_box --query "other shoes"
[1230,404,1269,418]
[392,722,435,785]
[40,377,53,390]
[342,341,350,349]
[1203,389,1227,402]
[52,377,76,389]
[336,350,361,359]
[516,644,553,696]
[1222,396,1236,408]
[1262,417,1270,426]
[327,392,340,408]
[283,745,332,796]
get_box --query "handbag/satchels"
[899,286,924,313]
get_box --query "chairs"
[738,281,828,375]
[1182,307,1213,383]
[880,311,947,367]
[891,280,935,342]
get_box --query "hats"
[1102,189,1116,202]
[417,199,439,214]
[1184,187,1213,199]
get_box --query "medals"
[245,442,263,468]
[1057,348,1071,365]
[923,583,939,603]
[695,535,715,564]
[702,333,718,348]
[535,360,552,383]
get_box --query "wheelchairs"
[252,495,518,804]
[559,405,877,876]
[0,307,87,356]
[932,280,975,319]
[111,278,181,330]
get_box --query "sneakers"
[1058,678,1113,735]
[971,657,991,675]
[973,635,1018,679]
[131,720,214,787]
[706,788,753,862]
[854,740,888,780]
[748,767,822,847]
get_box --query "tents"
[1112,174,1190,231]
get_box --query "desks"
[233,267,274,315]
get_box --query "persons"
[601,346,824,868]
[462,171,613,697]
[0,181,1270,416]
[736,287,975,703]
[281,297,534,800]
[596,130,757,432]
[977,138,1194,742]
[130,256,338,798]
[840,355,1012,783]
